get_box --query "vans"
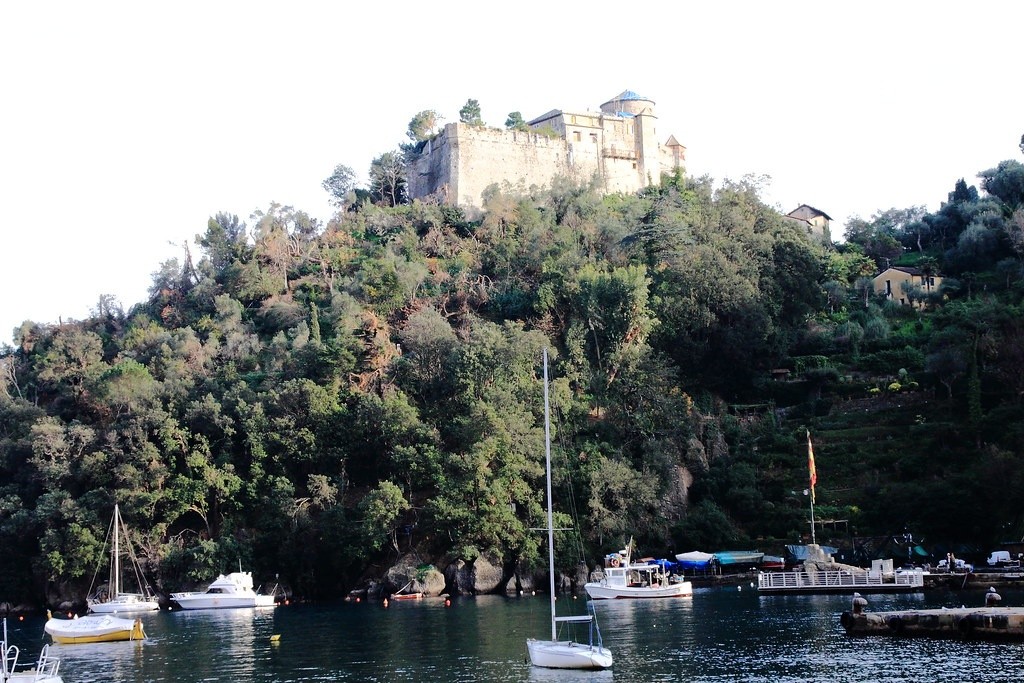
[986,550,1011,567]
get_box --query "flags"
[809,439,817,502]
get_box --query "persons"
[947,553,958,574]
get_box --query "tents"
[644,545,837,573]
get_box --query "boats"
[0,618,65,683]
[170,557,281,610]
[44,608,148,646]
[583,534,693,600]
[390,576,424,600]
[630,544,840,584]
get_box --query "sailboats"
[526,345,614,670]
[85,505,160,614]
